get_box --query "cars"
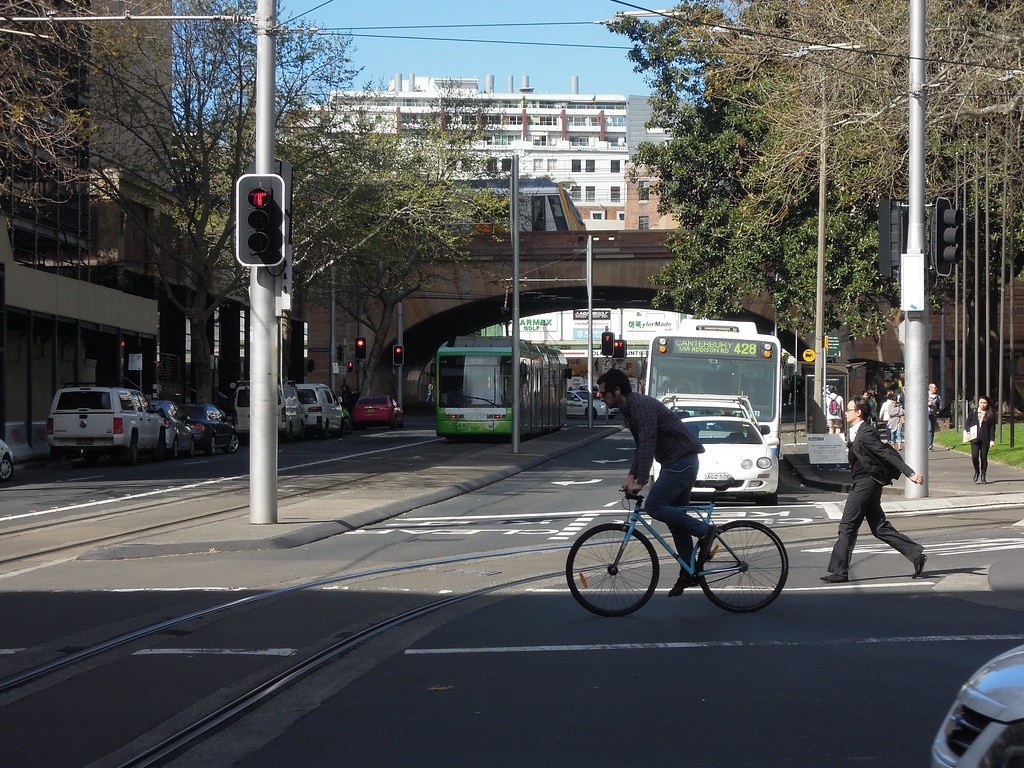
[929,644,1024,768]
[649,393,780,506]
[148,399,195,460]
[567,390,619,420]
[351,394,404,431]
[177,404,241,456]
[0,439,16,482]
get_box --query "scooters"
[342,407,350,434]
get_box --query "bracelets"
[909,473,914,477]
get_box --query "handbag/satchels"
[899,407,905,424]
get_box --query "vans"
[295,383,343,441]
[234,381,306,443]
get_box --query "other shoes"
[668,572,694,598]
[898,447,902,450]
[697,525,717,564]
[929,445,933,452]
[820,573,848,582]
[911,554,926,578]
[973,472,979,482]
[981,475,986,485]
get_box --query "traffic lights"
[355,337,366,360]
[612,340,626,359]
[934,197,961,276]
[602,332,614,356]
[337,346,343,361]
[348,361,353,372]
[393,345,404,366]
[235,174,286,267]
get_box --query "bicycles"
[565,478,789,618]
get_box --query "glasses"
[844,408,858,414]
[600,391,608,397]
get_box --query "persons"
[862,380,905,428]
[597,368,717,599]
[342,385,352,416]
[1002,392,1021,414]
[790,377,794,405]
[888,395,905,451]
[350,386,360,402]
[928,384,942,453]
[827,386,844,434]
[965,397,996,484]
[879,394,893,443]
[820,397,927,583]
[426,384,434,401]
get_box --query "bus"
[424,178,588,231]
[430,277,572,442]
[644,319,782,459]
[577,384,601,399]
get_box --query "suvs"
[45,382,166,466]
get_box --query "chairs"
[727,432,746,440]
[688,425,700,439]
[674,410,689,417]
